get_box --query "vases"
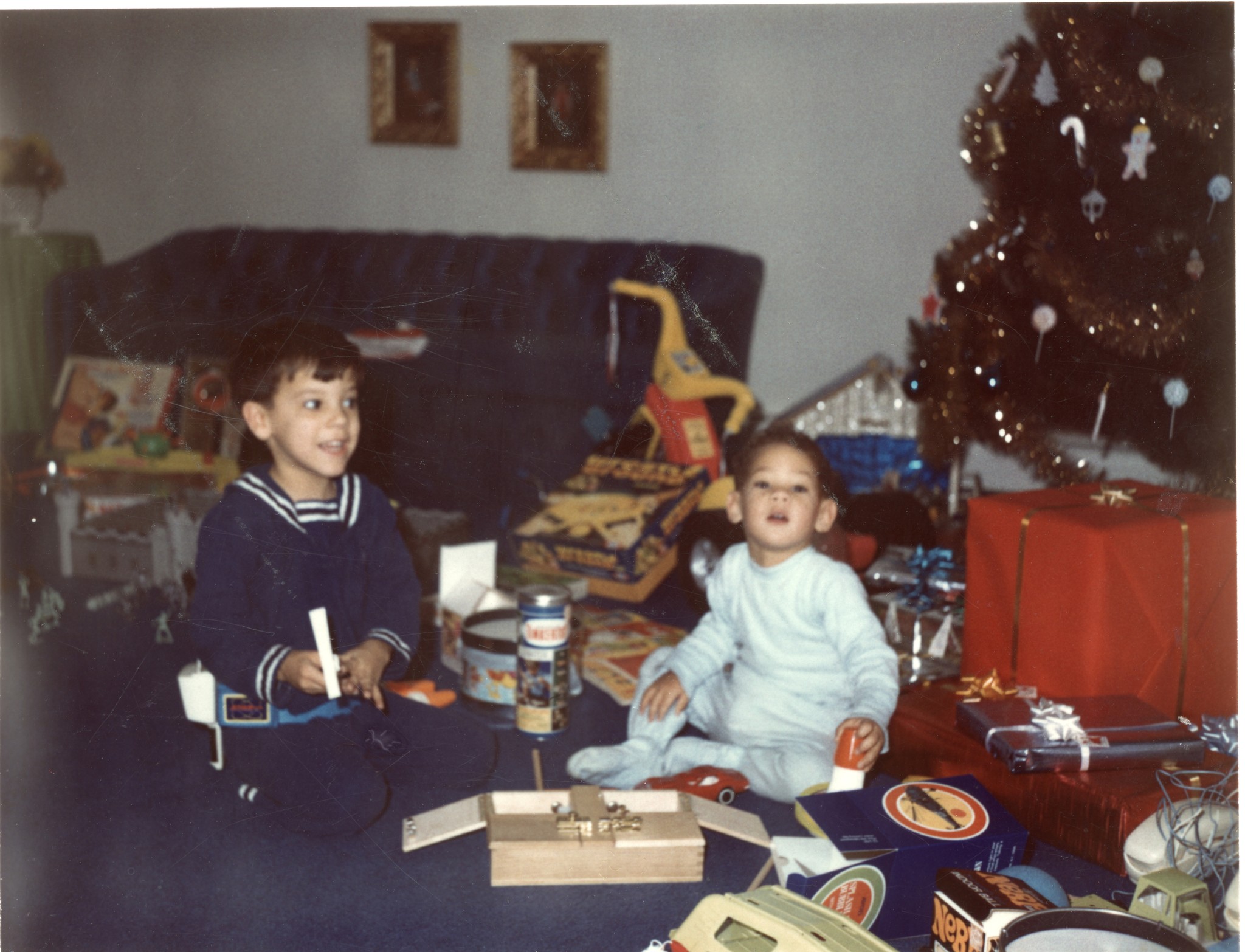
[0,183,45,238]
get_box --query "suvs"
[658,882,900,952]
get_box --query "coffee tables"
[0,233,102,436]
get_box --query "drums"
[985,905,1212,952]
[456,605,585,722]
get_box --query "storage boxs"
[963,474,1238,724]
[1020,746,1239,878]
[770,774,1035,941]
[929,869,1059,951]
[955,694,1205,772]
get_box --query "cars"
[631,764,750,806]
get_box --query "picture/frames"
[509,41,609,172]
[366,20,460,149]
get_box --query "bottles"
[517,578,570,739]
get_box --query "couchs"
[45,224,766,579]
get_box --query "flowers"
[1,131,66,191]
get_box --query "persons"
[569,425,899,807]
[186,317,422,711]
